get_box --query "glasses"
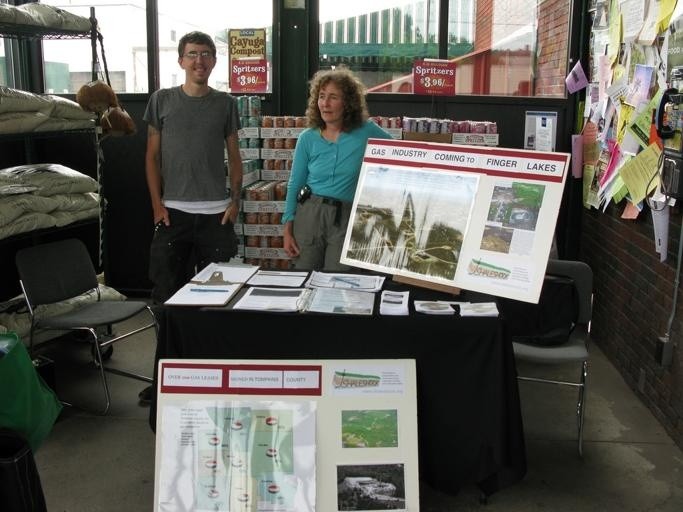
[183,51,214,59]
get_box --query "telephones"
[656,66,683,160]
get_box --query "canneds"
[224,96,498,271]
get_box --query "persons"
[281,67,395,272]
[138,31,243,403]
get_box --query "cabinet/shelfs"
[0,0,109,350]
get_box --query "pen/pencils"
[190,288,229,293]
[333,276,359,287]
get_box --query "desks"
[155,263,518,506]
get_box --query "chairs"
[11,237,162,417]
[516,258,598,465]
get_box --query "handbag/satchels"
[0,331,63,456]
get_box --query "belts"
[323,197,343,228]
